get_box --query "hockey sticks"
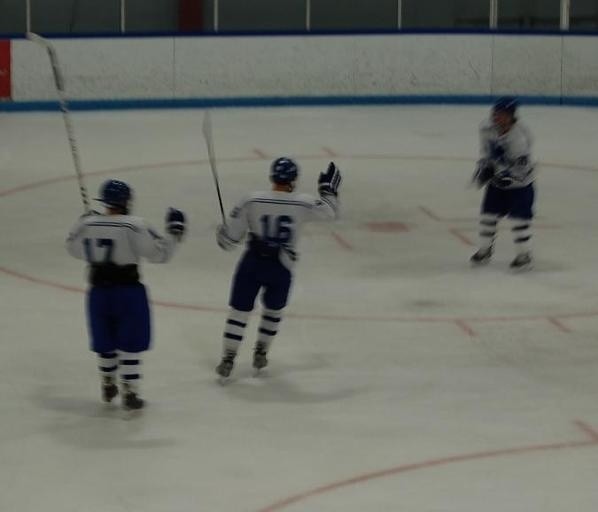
[27,31,90,212]
[201,110,225,222]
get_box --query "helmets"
[270,156,297,183]
[494,96,518,119]
[100,180,129,208]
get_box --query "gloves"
[164,208,185,243]
[475,160,496,187]
[317,163,341,199]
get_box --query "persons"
[57,177,185,412]
[464,96,537,269]
[214,155,344,379]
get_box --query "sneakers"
[469,245,493,262]
[123,382,143,410]
[252,345,268,370]
[102,379,118,404]
[508,252,532,268]
[214,352,238,378]
